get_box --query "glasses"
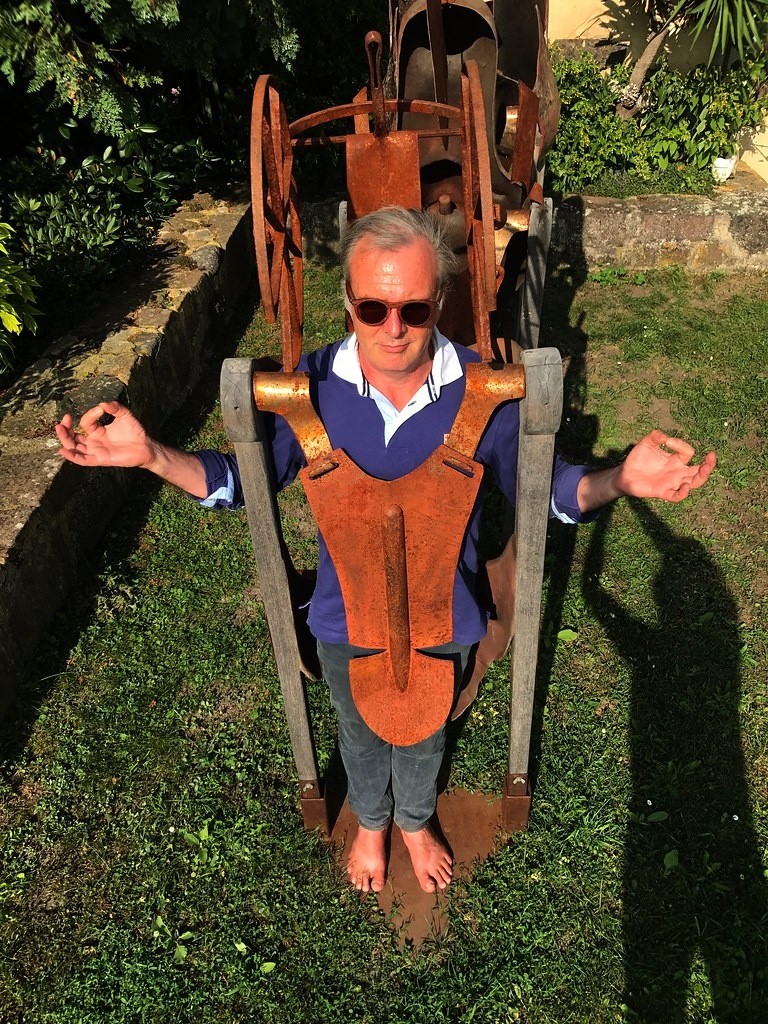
[345,278,446,327]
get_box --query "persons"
[55,206,716,895]
